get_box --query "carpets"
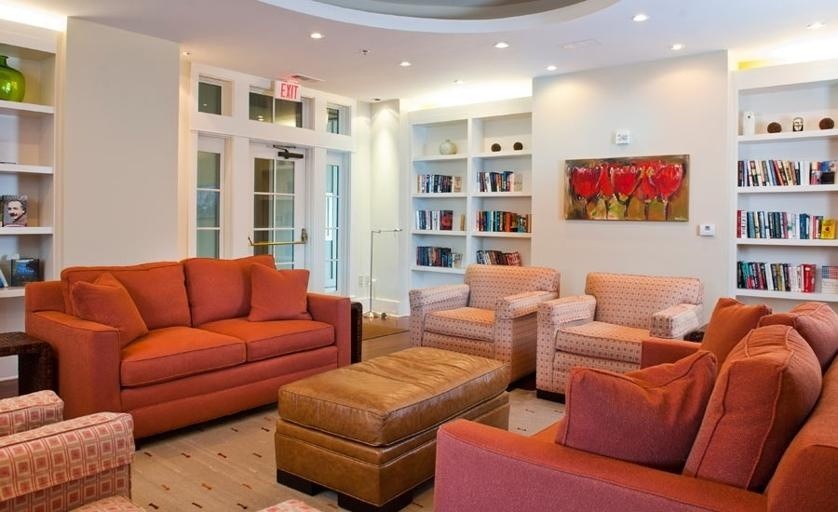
[362,322,409,341]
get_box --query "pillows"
[555,351,718,465]
[179,254,277,323]
[703,297,771,370]
[61,262,191,327]
[71,272,147,345]
[681,324,822,489]
[755,302,838,367]
[248,262,314,320]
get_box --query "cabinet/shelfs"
[0,19,60,376]
[733,60,837,307]
[410,95,532,273]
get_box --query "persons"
[7,200,27,226]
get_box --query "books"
[737,260,838,293]
[416,246,463,269]
[415,209,466,231]
[479,172,514,192]
[417,174,461,193]
[738,159,836,187]
[476,249,523,267]
[475,210,531,233]
[737,210,836,239]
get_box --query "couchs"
[69,494,321,512]
[0,389,135,512]
[273,344,509,512]
[410,263,560,379]
[23,280,352,437]
[432,339,836,512]
[535,271,704,400]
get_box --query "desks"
[0,331,56,393]
